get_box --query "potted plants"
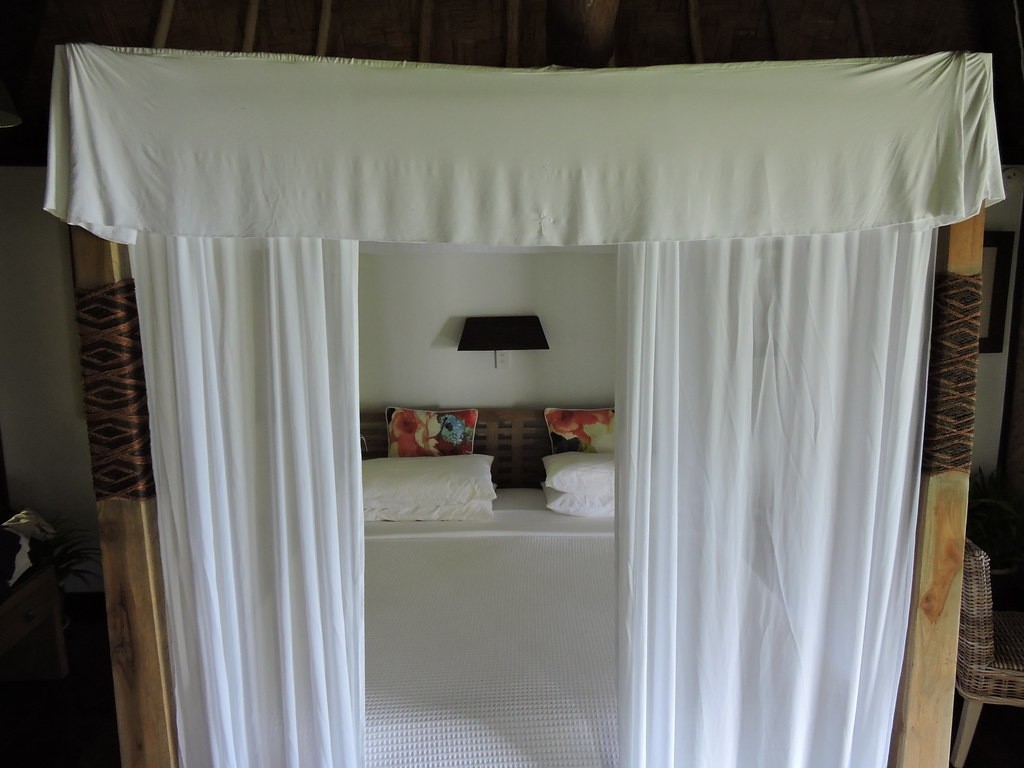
[966,467,1024,611]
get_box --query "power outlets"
[495,350,509,369]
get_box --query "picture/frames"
[979,230,1016,353]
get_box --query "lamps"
[457,315,549,350]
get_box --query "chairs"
[949,539,1024,768]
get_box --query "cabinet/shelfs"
[0,566,69,681]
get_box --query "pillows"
[541,482,615,517]
[542,452,614,495]
[362,454,498,522]
[544,407,616,455]
[385,407,479,457]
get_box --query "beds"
[43,43,1006,767]
